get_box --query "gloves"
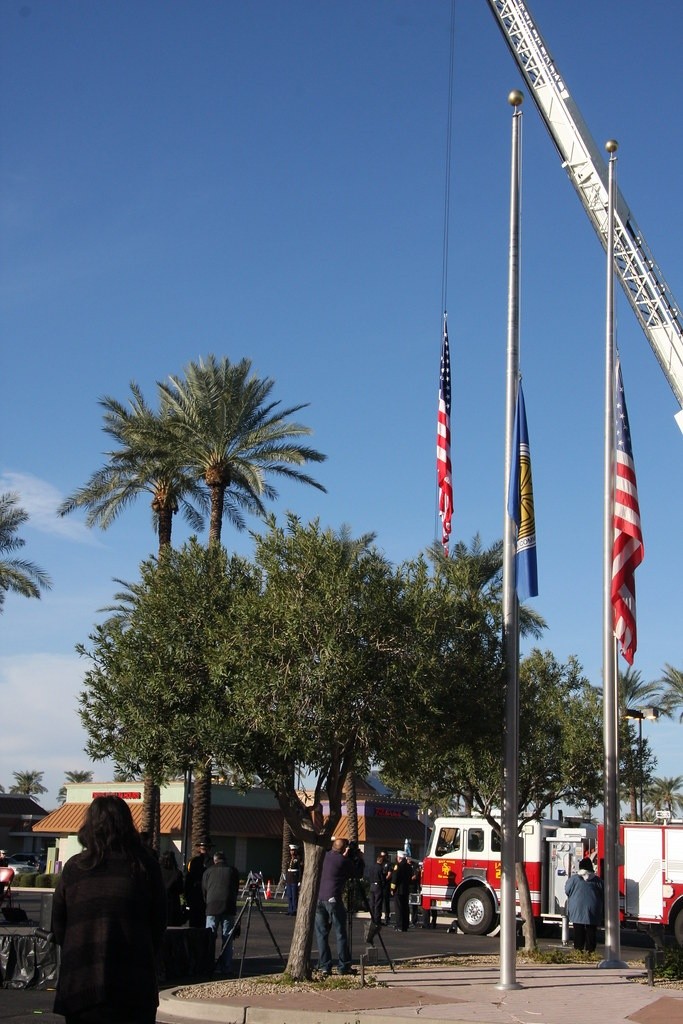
[285,886,287,889]
[297,882,301,886]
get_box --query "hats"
[397,850,407,858]
[195,836,216,846]
[289,845,298,850]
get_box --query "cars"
[5,853,39,876]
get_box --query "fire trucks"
[409,0,683,947]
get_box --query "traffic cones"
[264,881,273,899]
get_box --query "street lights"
[623,707,659,821]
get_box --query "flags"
[436,317,453,558]
[507,378,538,603]
[611,356,644,665]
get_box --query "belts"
[287,869,297,872]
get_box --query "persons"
[137,832,239,975]
[564,857,604,953]
[314,838,364,976]
[285,844,303,917]
[50,795,166,1024]
[369,850,437,932]
[0,850,8,868]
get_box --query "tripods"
[214,882,286,980]
[346,876,397,975]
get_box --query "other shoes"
[409,923,417,928]
[285,911,296,916]
[321,970,332,976]
[392,926,407,932]
[223,967,233,974]
[337,968,358,975]
